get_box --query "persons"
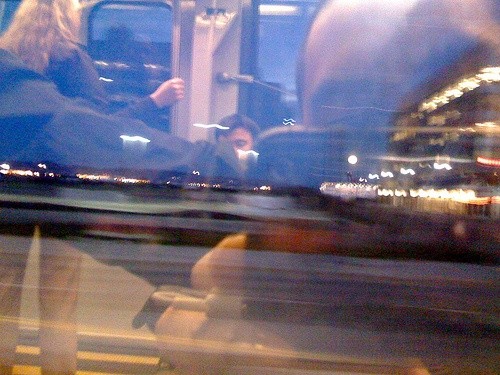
[0,0,187,374]
[212,112,259,157]
[187,1,499,374]
